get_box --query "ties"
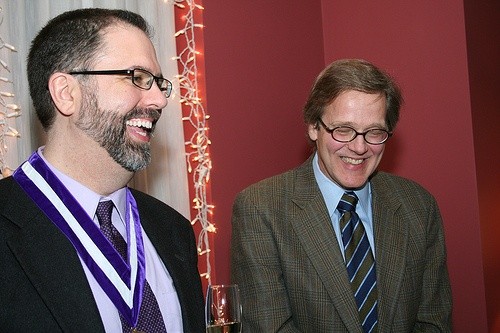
[336,191,378,333]
[96,200,167,333]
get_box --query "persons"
[0,7,213,333]
[230,59,452,332]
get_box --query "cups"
[204,284,242,333]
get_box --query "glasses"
[67,68,172,98]
[317,117,393,145]
